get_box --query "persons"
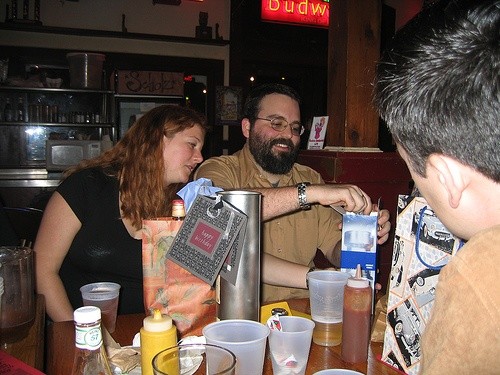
[34,105,207,322]
[363,0,500,375]
[193,85,391,306]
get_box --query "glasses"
[255,117,305,137]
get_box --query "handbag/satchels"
[379,194,468,375]
[141,217,217,340]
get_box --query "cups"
[0,246,36,344]
[312,369,364,375]
[266,316,315,375]
[202,319,270,375]
[151,343,238,375]
[307,270,352,347]
[79,282,121,334]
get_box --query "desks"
[0,293,411,375]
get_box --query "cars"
[414,208,454,244]
[411,274,440,297]
[390,302,423,358]
[389,237,405,289]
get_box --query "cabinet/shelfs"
[0,28,229,208]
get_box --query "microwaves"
[45,140,102,172]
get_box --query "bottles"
[0,96,100,124]
[140,309,179,375]
[341,263,372,363]
[71,305,112,375]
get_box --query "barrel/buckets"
[67,53,105,90]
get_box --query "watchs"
[295,182,312,211]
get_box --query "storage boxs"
[328,197,382,314]
